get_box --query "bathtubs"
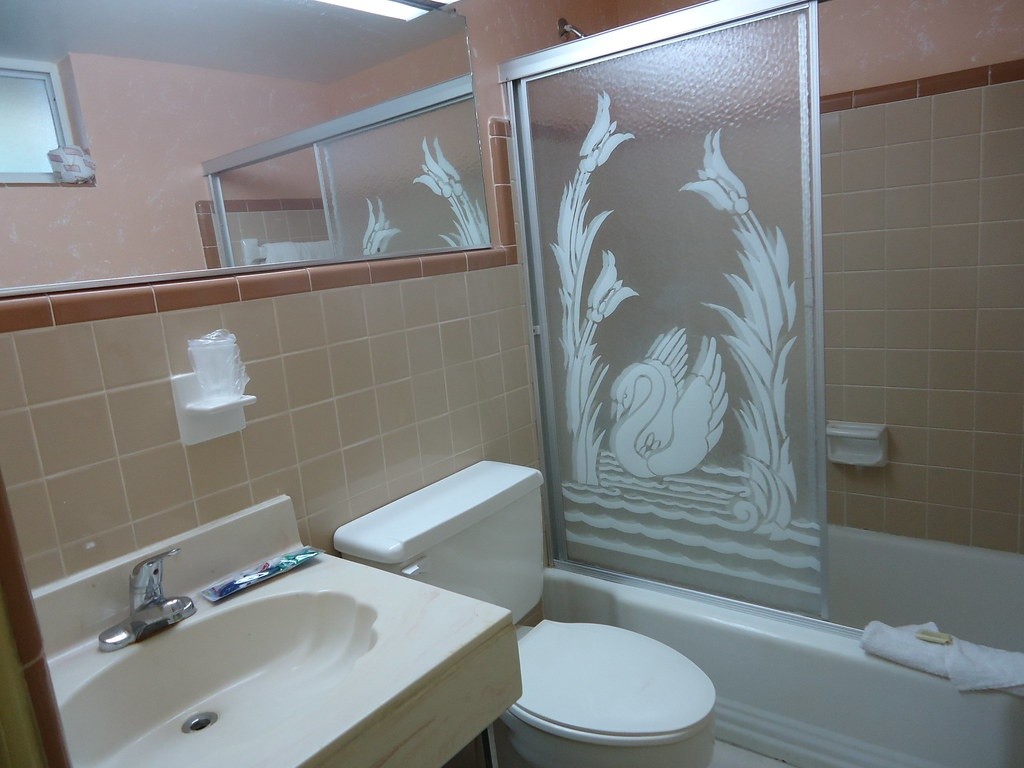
[542,521,1024,767]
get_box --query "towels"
[860,618,1024,698]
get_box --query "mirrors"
[1,0,494,301]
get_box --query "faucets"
[96,547,199,651]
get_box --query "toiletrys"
[202,544,325,603]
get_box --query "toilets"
[334,457,717,768]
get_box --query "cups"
[189,337,237,396]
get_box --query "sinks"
[59,591,380,765]
